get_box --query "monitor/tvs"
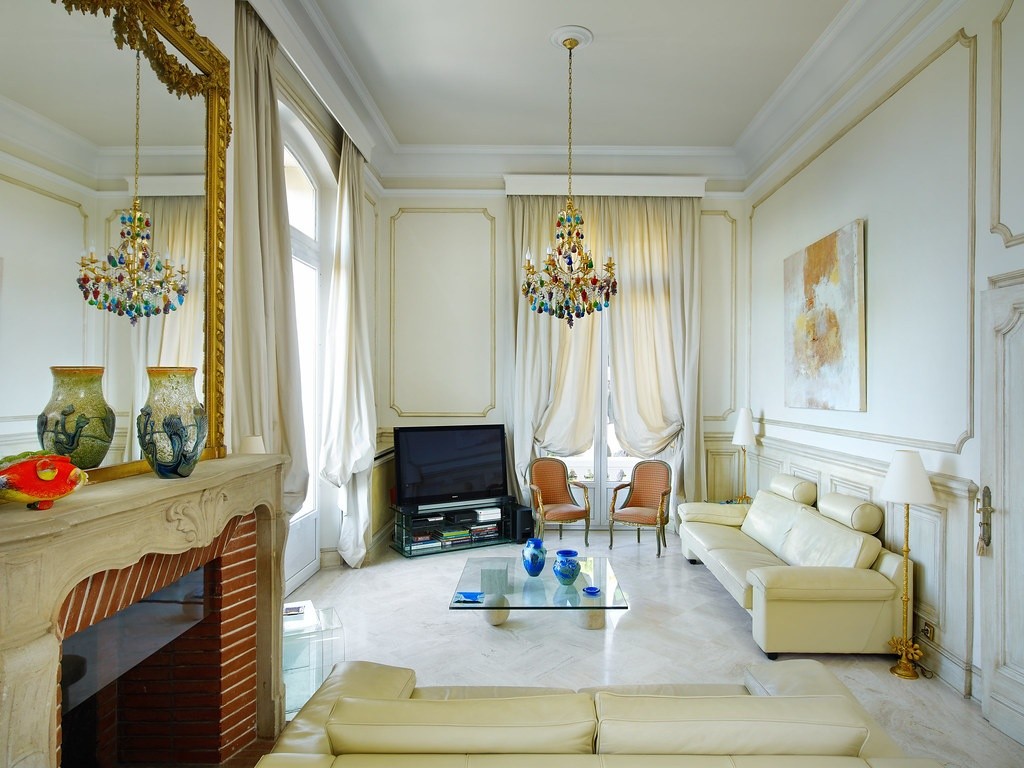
[393,423,509,510]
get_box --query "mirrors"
[0,0,236,497]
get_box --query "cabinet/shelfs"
[388,496,517,560]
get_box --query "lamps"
[77,49,186,323]
[879,450,934,681]
[731,408,757,504]
[522,24,617,327]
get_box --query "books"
[404,507,501,551]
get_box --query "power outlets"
[924,621,934,641]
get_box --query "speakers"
[510,501,534,541]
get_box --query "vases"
[553,550,582,585]
[37,366,116,471]
[522,538,547,577]
[137,366,210,479]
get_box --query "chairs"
[608,460,672,559]
[528,457,590,548]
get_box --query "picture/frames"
[784,218,867,412]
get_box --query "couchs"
[253,658,927,768]
[676,472,914,658]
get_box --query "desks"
[450,555,628,629]
[281,606,346,715]
[134,566,204,620]
